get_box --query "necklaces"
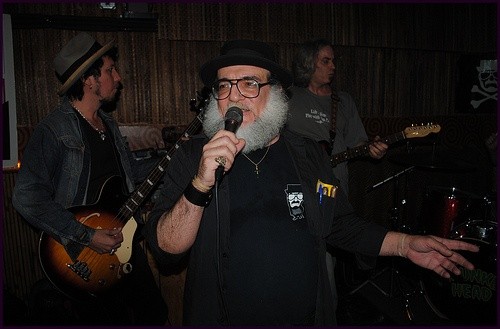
[242,145,270,174]
[70,101,105,141]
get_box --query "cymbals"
[370,162,417,192]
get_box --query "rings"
[215,156,226,166]
[112,249,114,252]
[110,251,113,255]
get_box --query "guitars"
[39,99,210,302]
[327,121,441,168]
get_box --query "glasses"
[211,78,273,100]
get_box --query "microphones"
[214,107,243,183]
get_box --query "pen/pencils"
[319,184,323,205]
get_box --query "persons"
[11,31,192,322]
[146,40,479,329]
[284,40,389,277]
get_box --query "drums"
[416,183,492,237]
[420,220,499,321]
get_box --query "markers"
[323,187,328,196]
[331,187,336,198]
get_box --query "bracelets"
[193,176,211,191]
[184,184,212,207]
[398,233,407,257]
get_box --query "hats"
[54,32,114,96]
[199,40,293,87]
